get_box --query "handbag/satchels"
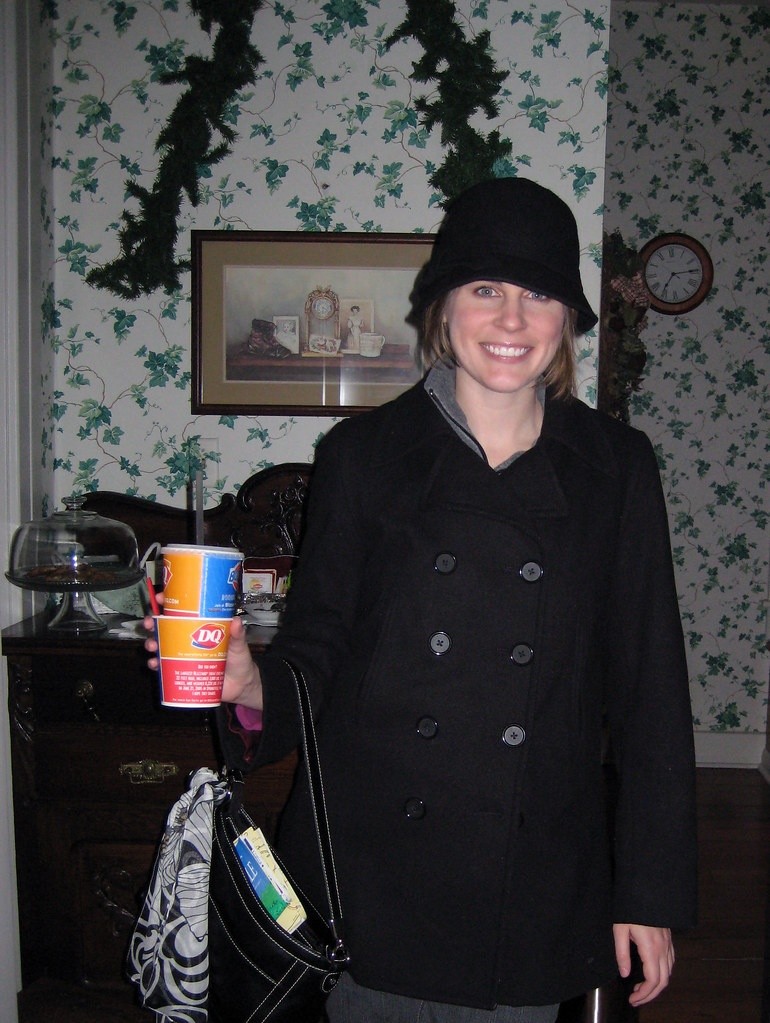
[130,645,352,1023]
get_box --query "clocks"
[642,232,713,317]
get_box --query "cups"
[150,614,233,708]
[160,544,245,618]
[359,333,386,358]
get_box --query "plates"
[4,562,145,631]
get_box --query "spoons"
[253,603,282,612]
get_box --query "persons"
[141,177,702,1023]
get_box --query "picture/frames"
[191,228,438,419]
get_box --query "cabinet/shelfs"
[0,461,314,1023]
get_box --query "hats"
[405,177,597,335]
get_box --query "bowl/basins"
[243,602,283,622]
